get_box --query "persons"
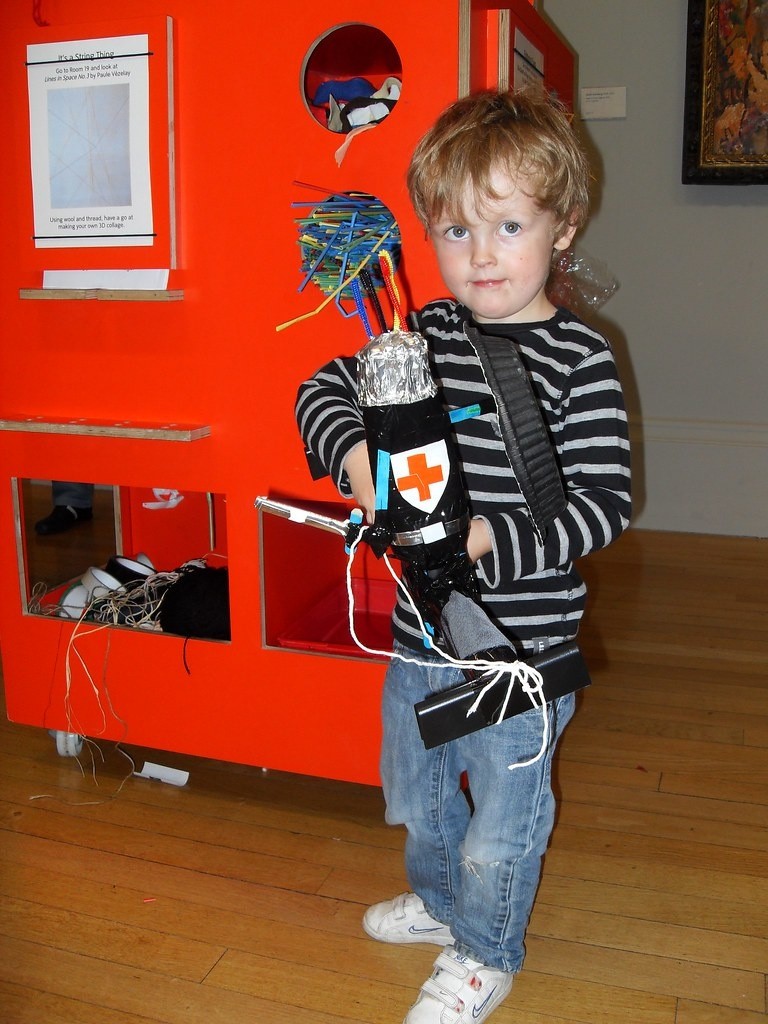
[294,88,632,1024]
[34,480,95,536]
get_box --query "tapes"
[81,566,128,600]
[56,580,89,618]
[104,552,158,585]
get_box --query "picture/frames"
[682,0,768,185]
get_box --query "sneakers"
[362,889,458,947]
[405,943,514,1024]
[34,504,94,535]
